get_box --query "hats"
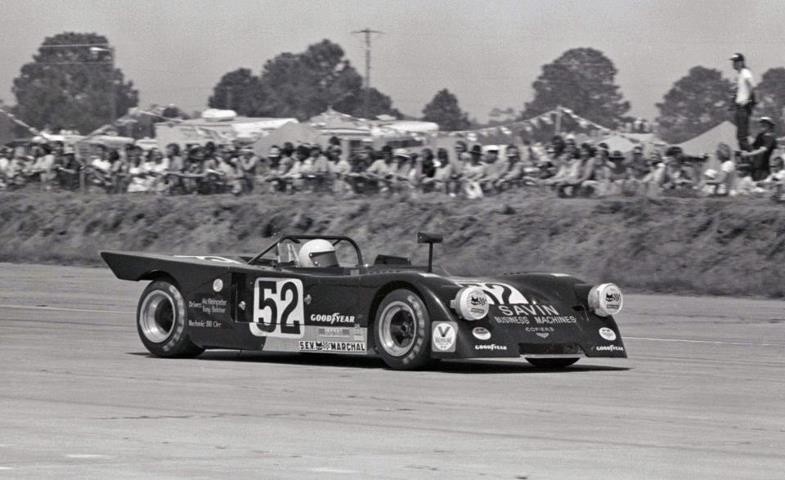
[729,53,745,62]
[394,149,410,159]
[498,132,752,177]
[268,148,281,159]
[486,144,500,152]
[758,116,775,125]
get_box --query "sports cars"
[99,232,626,369]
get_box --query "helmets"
[298,239,338,268]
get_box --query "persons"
[726,51,761,152]
[341,133,785,199]
[0,135,349,201]
[296,237,341,270]
[739,113,779,181]
